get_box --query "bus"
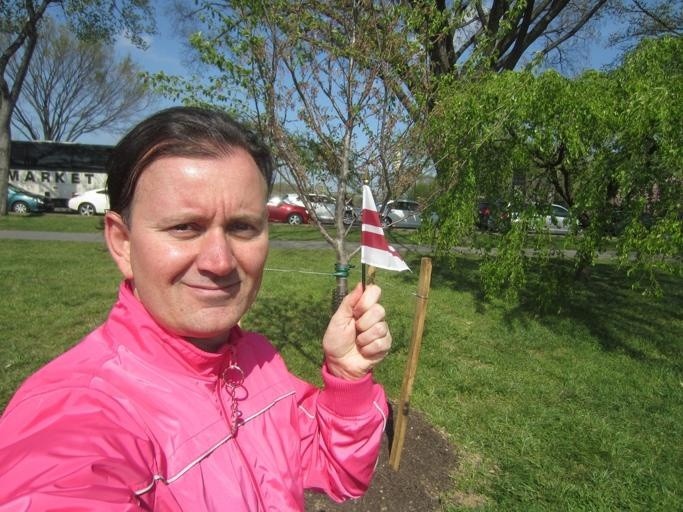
[8,141,116,206]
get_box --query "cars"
[508,201,583,236]
[281,194,357,225]
[378,199,445,229]
[5,182,53,217]
[267,198,310,226]
[67,187,112,217]
[475,201,513,233]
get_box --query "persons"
[1,104,396,512]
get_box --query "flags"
[358,182,413,273]
